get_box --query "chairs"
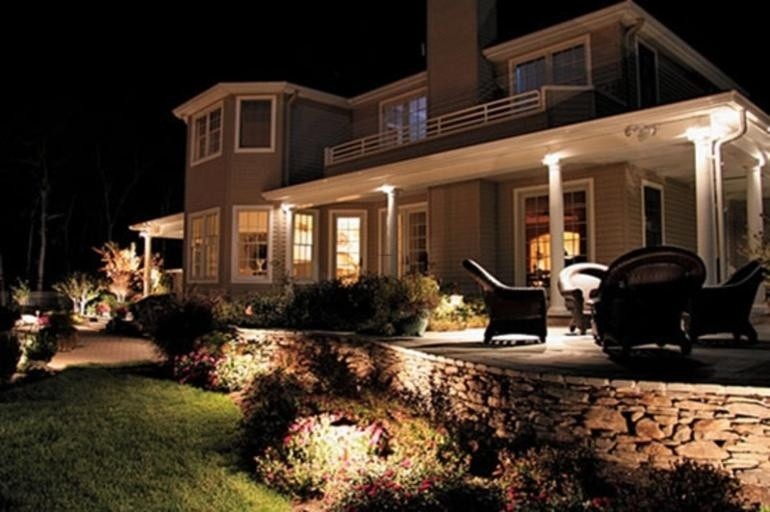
[704,260,766,341]
[588,245,706,361]
[558,262,608,334]
[461,256,548,347]
